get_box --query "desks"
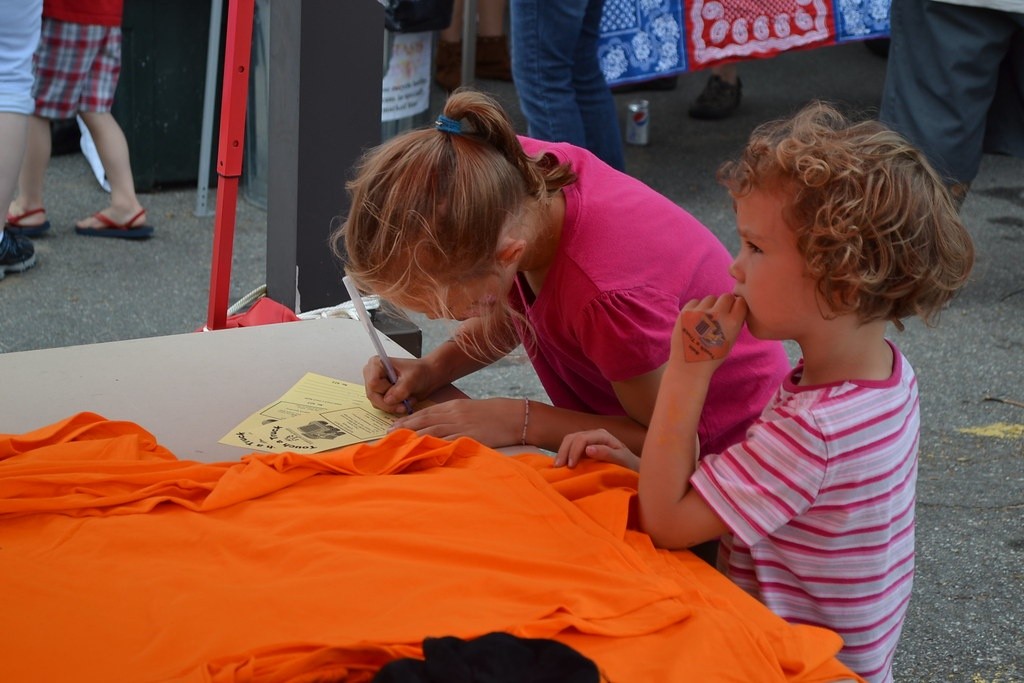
[0,319,855,683]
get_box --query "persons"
[511,0,743,174]
[328,91,794,570]
[865,0,1024,215]
[4,0,153,238]
[0,0,44,283]
[553,106,974,683]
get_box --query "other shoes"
[610,76,677,93]
[689,73,742,120]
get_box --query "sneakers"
[0,230,36,280]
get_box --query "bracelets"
[522,398,528,445]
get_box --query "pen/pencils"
[342,276,413,415]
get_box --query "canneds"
[625,99,649,145]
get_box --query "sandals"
[75,207,153,240]
[4,209,50,236]
